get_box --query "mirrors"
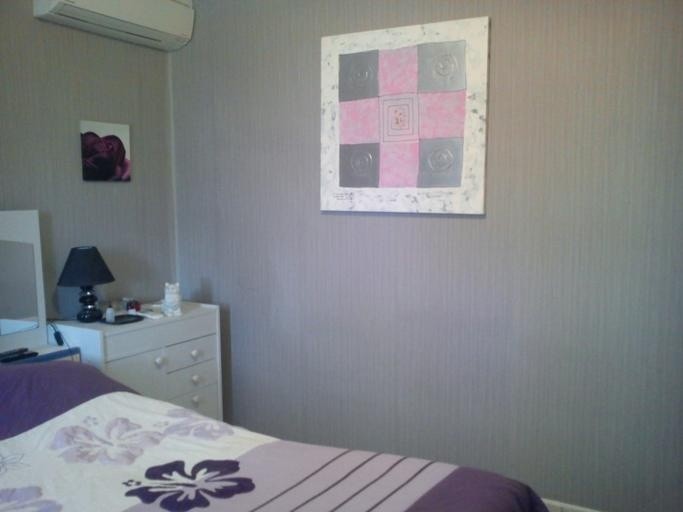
[0,240,38,336]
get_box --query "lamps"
[56,246,115,324]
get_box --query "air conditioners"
[31,0,195,52]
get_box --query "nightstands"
[19,343,81,363]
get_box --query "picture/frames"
[321,17,490,215]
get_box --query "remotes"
[0,347,29,358]
[0,351,39,365]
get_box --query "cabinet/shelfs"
[48,299,223,423]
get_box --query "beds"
[0,362,548,512]
